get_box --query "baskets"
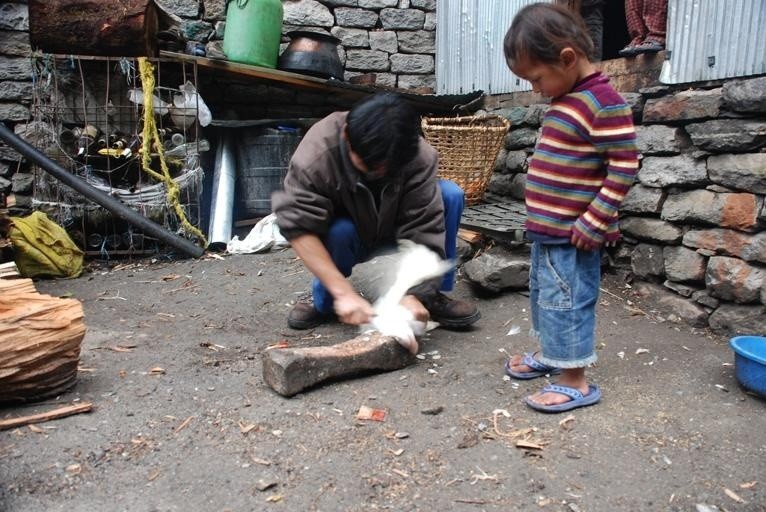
[421,114,511,206]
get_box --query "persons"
[504,1,640,413]
[618,1,666,55]
[268,92,480,330]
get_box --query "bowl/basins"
[728,335,766,398]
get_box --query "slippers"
[506,348,601,413]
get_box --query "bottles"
[77,124,99,160]
[98,127,144,162]
[157,126,189,140]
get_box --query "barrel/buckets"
[223,0,284,67]
[233,125,305,218]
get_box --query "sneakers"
[422,290,483,332]
[288,290,325,328]
